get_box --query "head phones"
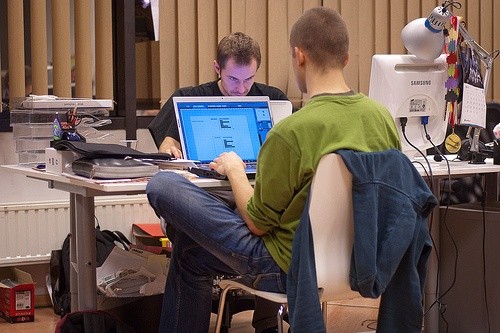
[458,138,494,161]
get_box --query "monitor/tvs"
[366,54,452,161]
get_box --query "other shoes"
[259,325,279,333]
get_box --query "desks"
[0,155,499,333]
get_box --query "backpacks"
[46,225,132,316]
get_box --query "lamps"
[400,0,500,164]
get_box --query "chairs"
[216,150,438,333]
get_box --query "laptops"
[171,96,276,180]
[270,99,293,128]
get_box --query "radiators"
[0,197,159,267]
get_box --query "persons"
[147,6,402,333]
[160,32,290,332]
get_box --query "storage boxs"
[0,267,37,324]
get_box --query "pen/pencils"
[51,103,84,131]
